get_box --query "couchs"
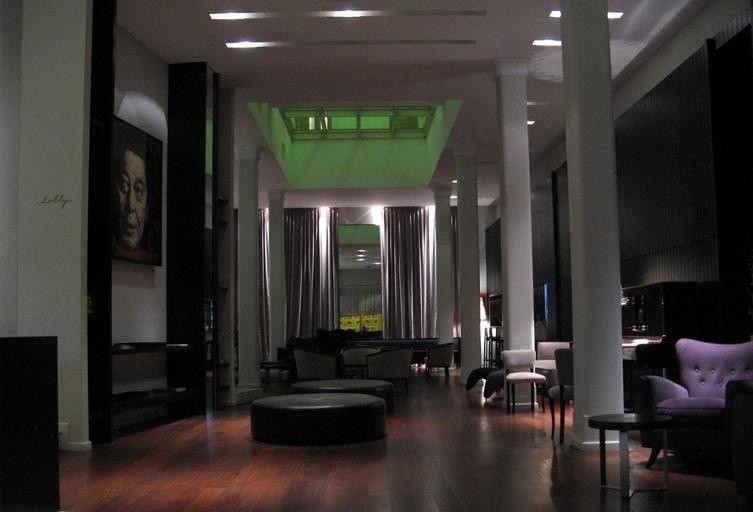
[342,337,436,367]
[288,335,322,353]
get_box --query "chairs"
[547,349,573,442]
[340,345,380,379]
[482,328,495,367]
[634,334,753,474]
[453,337,461,369]
[428,342,454,375]
[535,342,570,412]
[366,348,413,393]
[294,350,337,379]
[501,349,545,413]
[488,328,503,369]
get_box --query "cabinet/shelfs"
[112,342,189,415]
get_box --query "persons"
[111,141,151,248]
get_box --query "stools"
[290,379,393,414]
[250,394,387,445]
